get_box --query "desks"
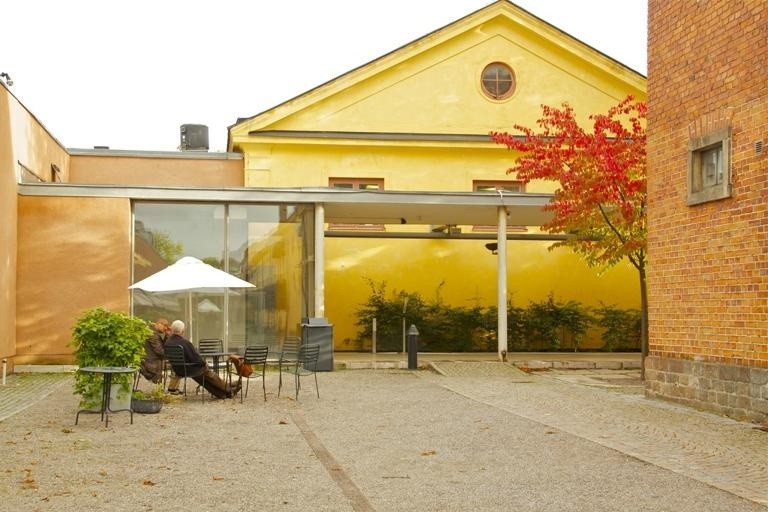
[74,366,136,428]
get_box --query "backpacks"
[229,353,253,377]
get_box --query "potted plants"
[71,308,168,414]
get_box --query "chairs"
[132,336,320,402]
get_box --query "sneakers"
[231,378,242,399]
[169,387,183,395]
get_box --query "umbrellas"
[125,255,258,345]
[147,288,242,298]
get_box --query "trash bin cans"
[300,317,334,372]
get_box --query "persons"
[140,319,185,395]
[165,319,242,401]
